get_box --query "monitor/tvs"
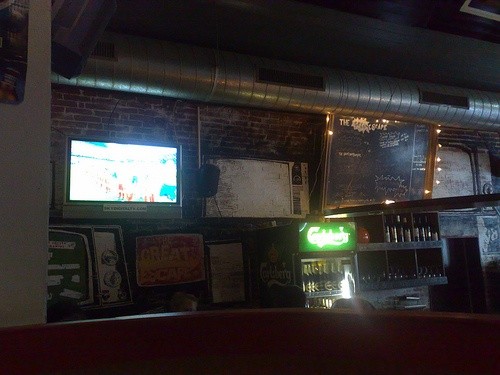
[63,136,184,220]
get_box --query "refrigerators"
[256,221,361,307]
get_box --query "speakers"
[196,164,220,197]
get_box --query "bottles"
[357,226,371,243]
[383,214,439,243]
[270,239,299,285]
[304,262,345,309]
[382,272,443,280]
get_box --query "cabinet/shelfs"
[296,210,447,312]
